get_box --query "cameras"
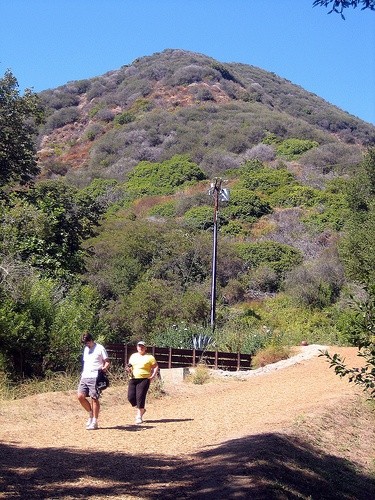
[128,364,132,368]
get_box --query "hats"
[138,341,147,346]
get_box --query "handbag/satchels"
[95,369,109,390]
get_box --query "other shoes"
[86,418,92,426]
[142,408,146,413]
[88,422,98,430]
[136,415,143,424]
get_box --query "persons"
[78,333,110,430]
[125,341,158,423]
